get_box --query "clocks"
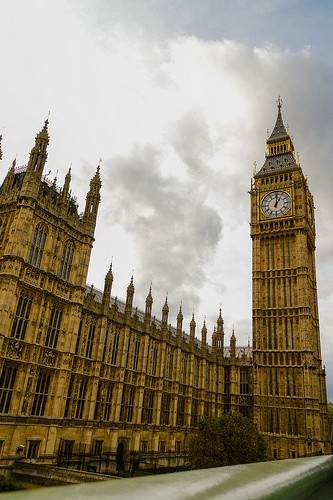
[260,190,292,220]
